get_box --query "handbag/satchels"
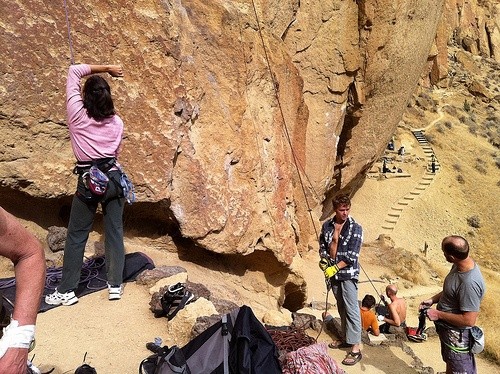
[137,305,281,374]
[466,325,487,355]
[86,165,107,194]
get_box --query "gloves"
[324,264,338,280]
[318,258,329,272]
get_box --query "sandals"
[329,338,353,348]
[340,350,362,365]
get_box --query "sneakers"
[43,290,78,306]
[106,281,121,300]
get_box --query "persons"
[367,284,407,334]
[0,206,47,374]
[416,235,485,374]
[45,63,134,307]
[321,294,380,349]
[318,194,364,365]
[397,146,406,164]
[430,154,436,172]
[383,164,395,173]
